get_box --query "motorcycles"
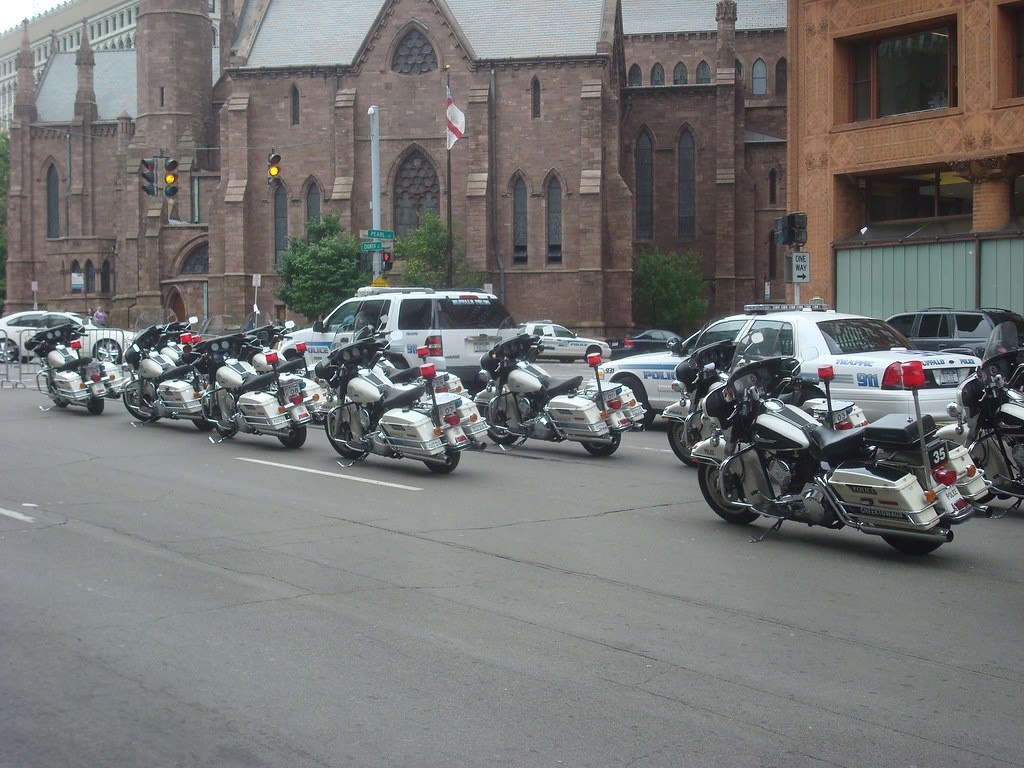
[471,312,649,458]
[690,326,994,556]
[931,320,1024,521]
[660,336,873,470]
[24,305,493,476]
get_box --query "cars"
[0,310,137,365]
[591,300,986,433]
[516,318,613,364]
[602,328,686,361]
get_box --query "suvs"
[881,306,1024,358]
[273,285,522,394]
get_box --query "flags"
[447,85,465,151]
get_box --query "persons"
[246,316,253,331]
[93,305,108,326]
[319,313,327,322]
[87,308,94,316]
[62,303,70,312]
[339,303,356,323]
[353,310,370,330]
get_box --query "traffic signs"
[791,252,811,282]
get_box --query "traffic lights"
[787,214,800,228]
[164,158,179,199]
[266,154,282,188]
[141,158,158,198]
[383,252,390,261]
[787,228,800,246]
